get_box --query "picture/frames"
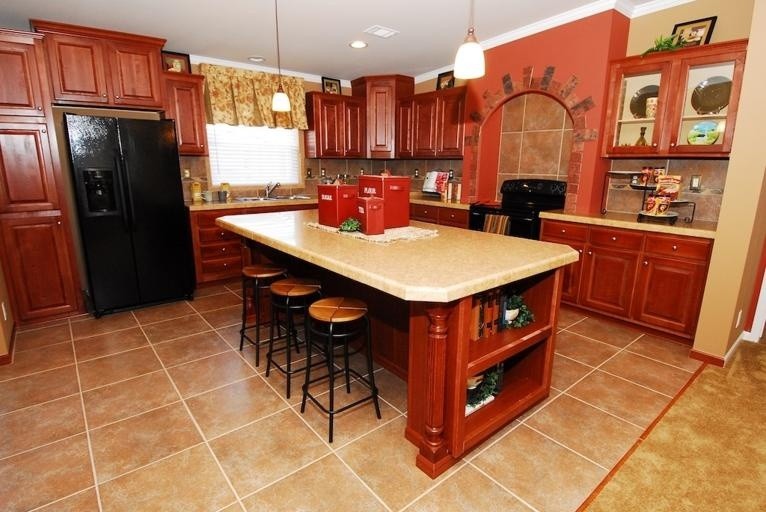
[669,16,717,45]
[161,50,191,73]
[437,70,454,89]
[321,76,341,94]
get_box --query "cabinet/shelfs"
[0,28,84,332]
[602,39,748,159]
[306,90,366,159]
[540,219,646,347]
[395,91,465,158]
[631,232,714,346]
[168,73,209,155]
[30,19,166,112]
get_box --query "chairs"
[483,214,510,236]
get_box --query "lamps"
[272,0,290,112]
[454,0,485,79]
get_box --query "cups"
[199,191,212,202]
[217,190,231,203]
[212,191,219,201]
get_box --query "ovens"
[469,205,542,243]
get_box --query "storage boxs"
[357,197,384,235]
[316,185,356,228]
[358,175,410,229]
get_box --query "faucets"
[265,183,280,198]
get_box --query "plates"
[670,199,687,203]
[686,120,720,145]
[608,170,641,174]
[639,210,679,217]
[690,75,732,113]
[630,183,656,188]
[629,84,659,118]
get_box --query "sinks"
[233,196,284,202]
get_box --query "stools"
[240,264,301,367]
[300,297,381,443]
[267,277,325,399]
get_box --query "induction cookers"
[470,198,563,221]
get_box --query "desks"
[215,210,580,480]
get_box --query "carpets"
[575,323,766,512]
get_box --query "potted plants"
[505,294,536,327]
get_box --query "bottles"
[221,183,229,204]
[445,170,458,202]
[182,169,193,202]
[635,127,648,145]
[192,181,203,204]
